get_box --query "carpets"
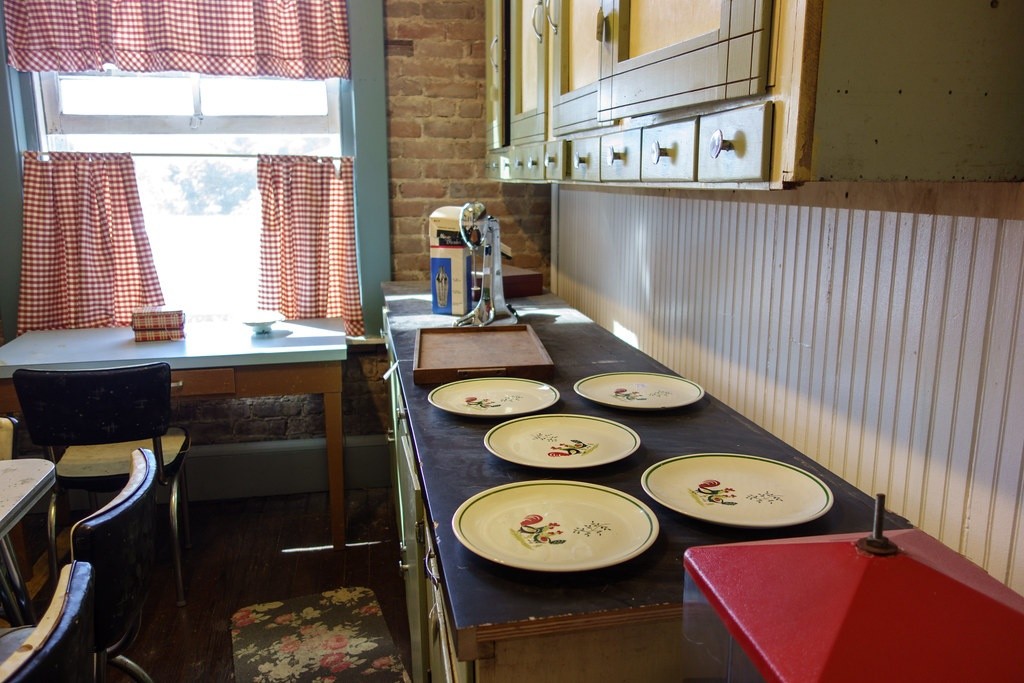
[230,586,412,683]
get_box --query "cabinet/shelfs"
[484,0,1024,219]
[381,276,907,683]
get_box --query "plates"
[641,452,833,529]
[428,377,560,420]
[452,479,659,574]
[484,413,642,468]
[572,371,706,411]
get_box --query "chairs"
[59,445,166,683]
[0,560,154,683]
[11,362,196,610]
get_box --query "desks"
[0,316,359,552]
[0,456,58,628]
